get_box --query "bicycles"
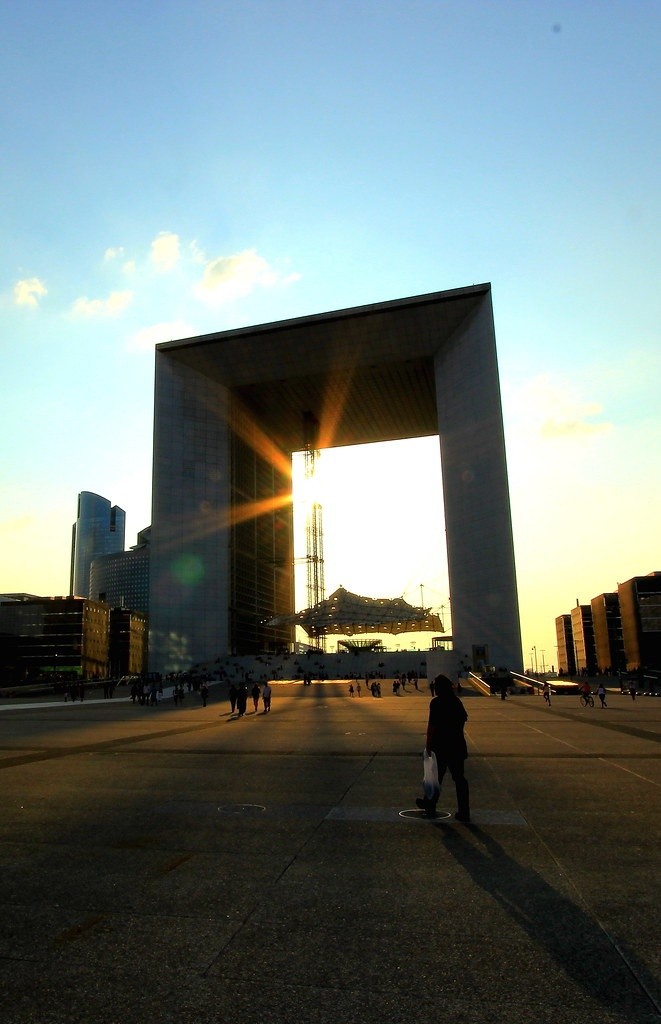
[580,689,595,707]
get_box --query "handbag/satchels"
[423,748,440,800]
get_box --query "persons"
[416,674,471,822]
[541,681,551,707]
[579,681,590,704]
[629,685,636,701]
[559,666,648,688]
[596,684,607,709]
[62,669,507,716]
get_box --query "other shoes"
[415,798,436,814]
[455,812,470,821]
